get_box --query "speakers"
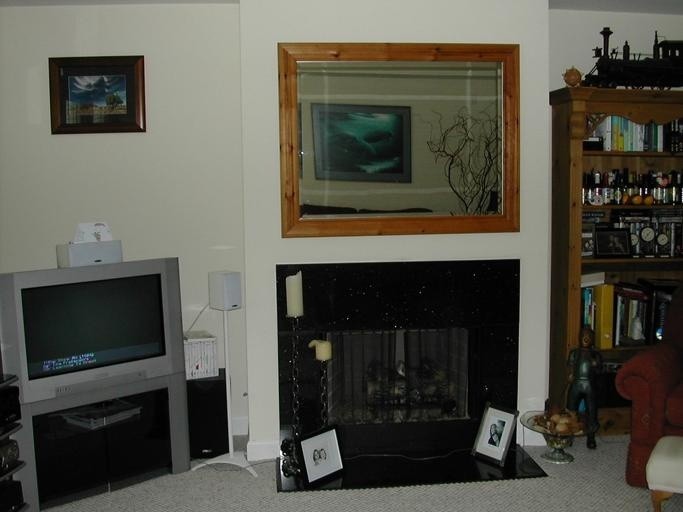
[208,270,242,310]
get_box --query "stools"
[646,436,683,512]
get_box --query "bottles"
[581,169,682,206]
[581,228,593,257]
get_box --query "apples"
[622,195,654,205]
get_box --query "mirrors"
[277,39,520,239]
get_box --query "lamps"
[190,269,261,477]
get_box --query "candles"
[286,270,304,317]
[307,341,332,359]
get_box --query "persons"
[567,326,603,449]
[488,420,505,448]
[608,233,625,254]
[313,448,327,465]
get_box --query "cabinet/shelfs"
[548,87,681,450]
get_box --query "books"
[64,400,143,430]
[580,270,680,351]
[587,113,683,153]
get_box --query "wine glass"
[518,409,600,465]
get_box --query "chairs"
[616,342,682,490]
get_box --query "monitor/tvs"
[0,257,185,404]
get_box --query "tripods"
[190,312,257,477]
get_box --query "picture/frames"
[47,55,146,134]
[592,228,632,259]
[293,425,345,488]
[469,402,519,467]
[309,100,412,184]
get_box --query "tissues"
[55,220,123,266]
